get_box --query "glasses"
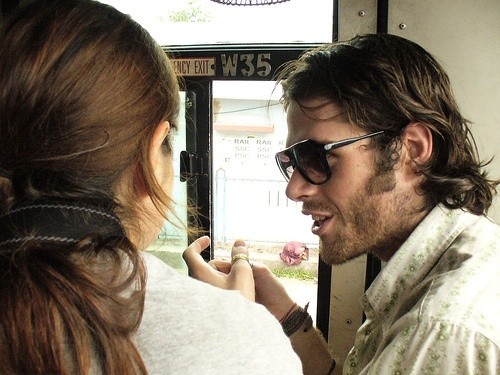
[274,129,387,184]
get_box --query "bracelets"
[328,358,336,375]
[280,303,312,337]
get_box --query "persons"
[183,32,499,375]
[0,0,304,374]
